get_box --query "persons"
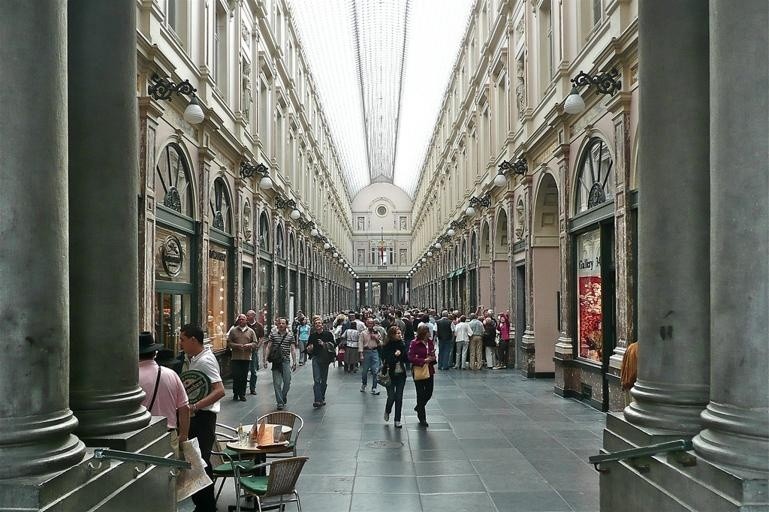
[227,305,510,428]
[139,333,190,452]
[178,323,226,511]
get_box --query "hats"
[139,331,164,355]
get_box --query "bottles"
[238,422,244,432]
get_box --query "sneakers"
[371,387,380,396]
[384,411,389,421]
[395,420,402,428]
[250,390,256,394]
[414,406,428,426]
[360,384,366,392]
[438,362,507,370]
[277,403,288,410]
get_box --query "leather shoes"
[313,400,328,408]
[233,394,246,401]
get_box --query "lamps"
[405,215,469,279]
[564,66,621,115]
[148,72,204,124]
[298,218,359,279]
[494,158,529,186]
[275,196,300,219]
[240,160,272,189]
[466,192,493,216]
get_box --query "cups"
[238,432,248,446]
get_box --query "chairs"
[209,411,309,512]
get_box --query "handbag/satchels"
[377,356,391,386]
[268,345,282,361]
[413,364,430,381]
